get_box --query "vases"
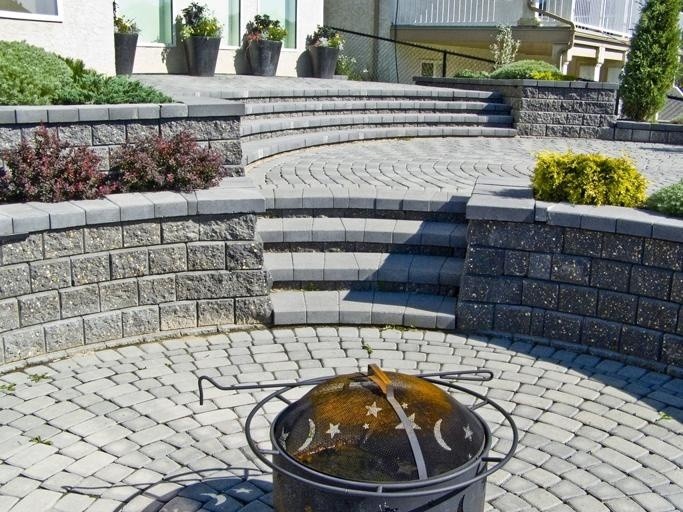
[247,39,282,77]
[308,47,339,79]
[114,33,137,77]
[182,37,220,77]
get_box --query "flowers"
[113,2,143,34]
[307,23,346,52]
[242,15,288,56]
[175,1,220,49]
